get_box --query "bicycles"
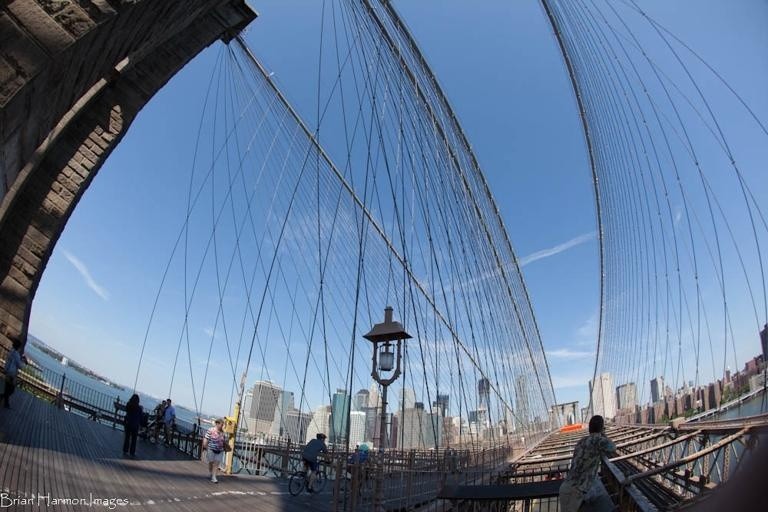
[332,467,375,502]
[287,460,329,496]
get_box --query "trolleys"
[136,413,159,443]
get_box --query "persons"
[347,444,369,497]
[121,391,141,456]
[301,431,332,492]
[202,418,230,483]
[443,441,469,474]
[558,413,616,511]
[162,398,176,447]
[153,398,167,443]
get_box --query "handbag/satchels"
[223,441,232,452]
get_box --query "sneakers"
[211,478,218,483]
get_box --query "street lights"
[361,306,413,512]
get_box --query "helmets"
[317,433,327,440]
[359,445,369,451]
